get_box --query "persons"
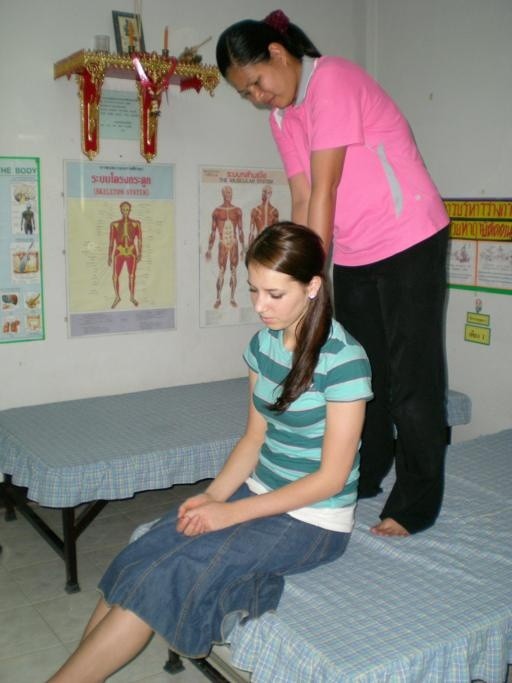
[48,221,375,683]
[215,9,451,537]
[108,201,142,309]
[21,205,35,235]
[248,185,279,248]
[205,186,245,308]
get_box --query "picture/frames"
[111,9,146,54]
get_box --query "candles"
[163,25,168,49]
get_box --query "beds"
[1,376,475,595]
[129,424,511,683]
